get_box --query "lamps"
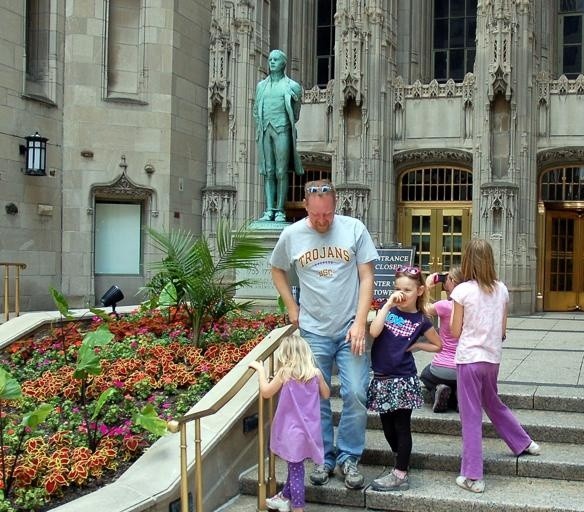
[17,130,51,178]
[99,283,126,315]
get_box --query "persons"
[449,239,541,493]
[248,334,330,512]
[270,180,379,490]
[366,266,442,492]
[253,49,306,222]
[425,266,466,411]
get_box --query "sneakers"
[308,462,334,485]
[392,452,409,470]
[432,383,452,413]
[371,469,409,491]
[336,456,365,489]
[455,475,486,493]
[265,491,290,512]
[524,439,541,456]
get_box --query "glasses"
[307,186,333,194]
[394,265,422,276]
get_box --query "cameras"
[434,274,448,284]
